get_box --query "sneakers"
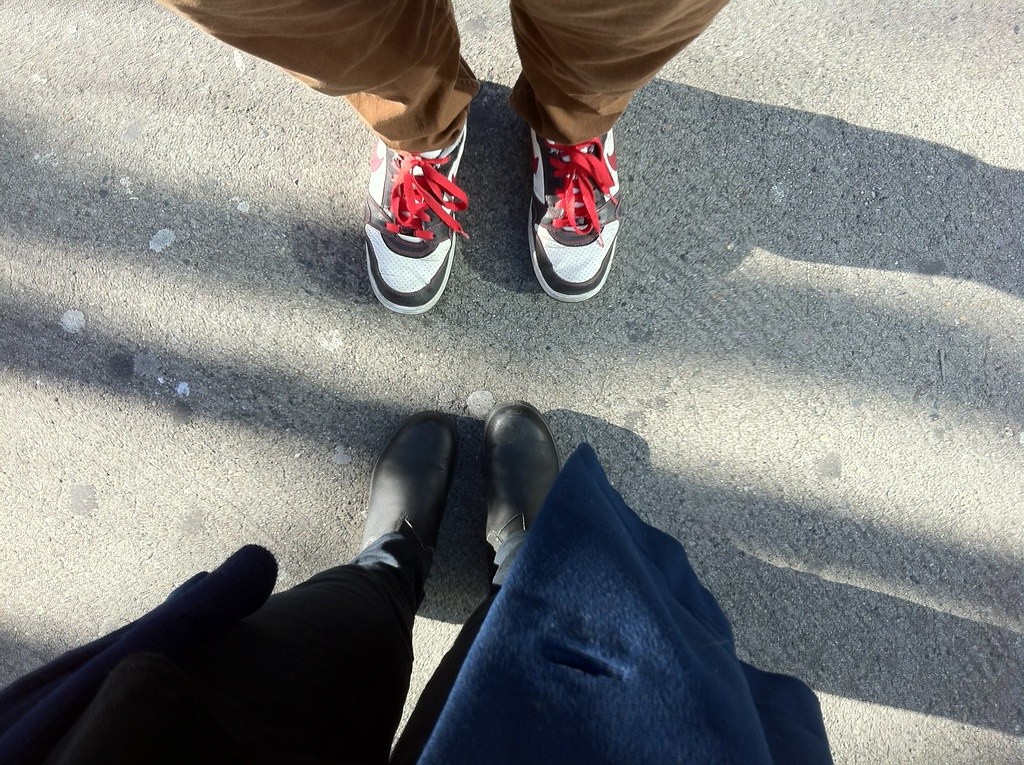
[362,118,468,314]
[529,126,621,304]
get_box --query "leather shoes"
[359,410,457,574]
[483,402,560,560]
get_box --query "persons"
[0,398,837,765]
[158,0,735,317]
